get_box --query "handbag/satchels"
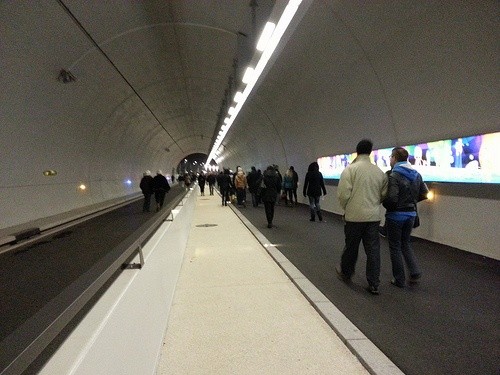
[413,215,420,228]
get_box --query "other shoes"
[407,272,423,283]
[378,226,386,237]
[369,284,379,293]
[391,280,405,287]
[336,266,354,282]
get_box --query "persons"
[384,147,429,289]
[207,173,216,196]
[140,170,155,212]
[199,175,205,196]
[378,158,412,240]
[217,166,263,209]
[178,172,197,186]
[304,162,326,221]
[273,163,298,207]
[152,170,170,208]
[259,167,281,228]
[336,140,389,294]
